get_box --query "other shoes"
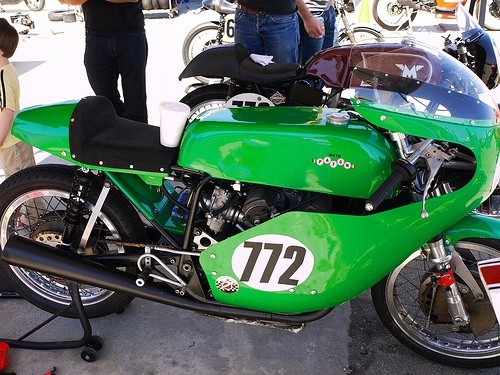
[13,215,40,230]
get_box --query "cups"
[159,101,190,147]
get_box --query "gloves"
[250,54,273,67]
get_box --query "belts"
[236,3,264,16]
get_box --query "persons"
[0,18,48,230]
[297,0,338,65]
[234,0,300,64]
[58,0,150,125]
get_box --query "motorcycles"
[0,1,500,368]
